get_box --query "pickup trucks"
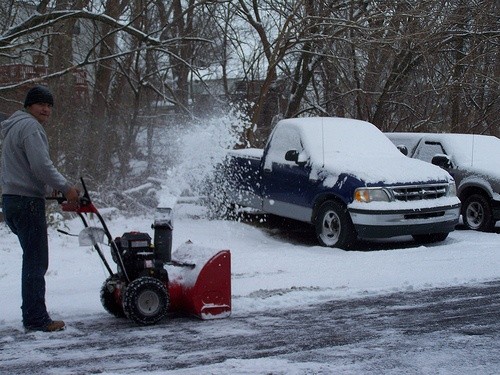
[203,116,461,251]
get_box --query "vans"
[382,132,500,233]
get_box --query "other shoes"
[47,321,66,331]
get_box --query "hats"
[23,86,54,108]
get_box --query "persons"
[1,85,82,334]
